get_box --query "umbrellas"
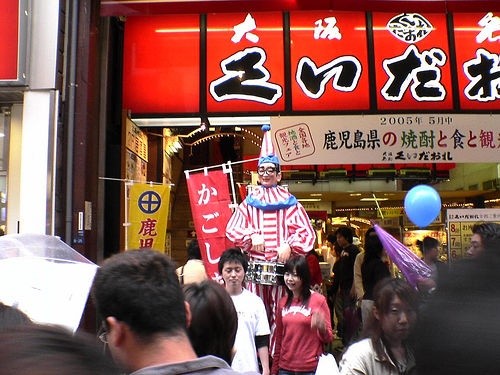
[0,234,101,332]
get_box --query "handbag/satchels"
[314,334,339,375]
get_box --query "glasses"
[96,325,110,343]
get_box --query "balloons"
[404,184,441,229]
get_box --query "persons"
[331,227,359,293]
[181,280,238,368]
[359,235,392,336]
[216,247,271,375]
[351,229,392,304]
[368,218,433,292]
[416,236,449,287]
[175,239,209,286]
[338,278,419,375]
[418,221,499,296]
[88,248,261,375]
[269,254,332,375]
[224,125,317,370]
[306,248,326,296]
[326,234,342,283]
